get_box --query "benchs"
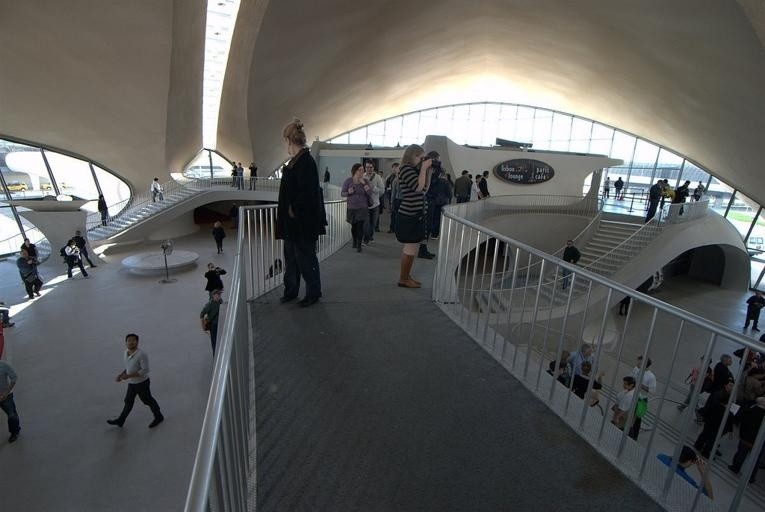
[121,251,199,276]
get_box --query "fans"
[159,238,175,283]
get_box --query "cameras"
[422,151,442,167]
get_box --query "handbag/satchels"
[364,178,373,207]
[584,389,599,407]
[702,369,713,393]
[636,400,648,418]
[26,272,38,284]
[203,316,210,332]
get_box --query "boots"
[418,244,435,257]
[398,254,421,288]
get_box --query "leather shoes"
[36,292,40,296]
[29,295,34,298]
[107,418,123,427]
[280,296,293,304]
[8,431,17,442]
[149,414,164,428]
[299,294,318,306]
